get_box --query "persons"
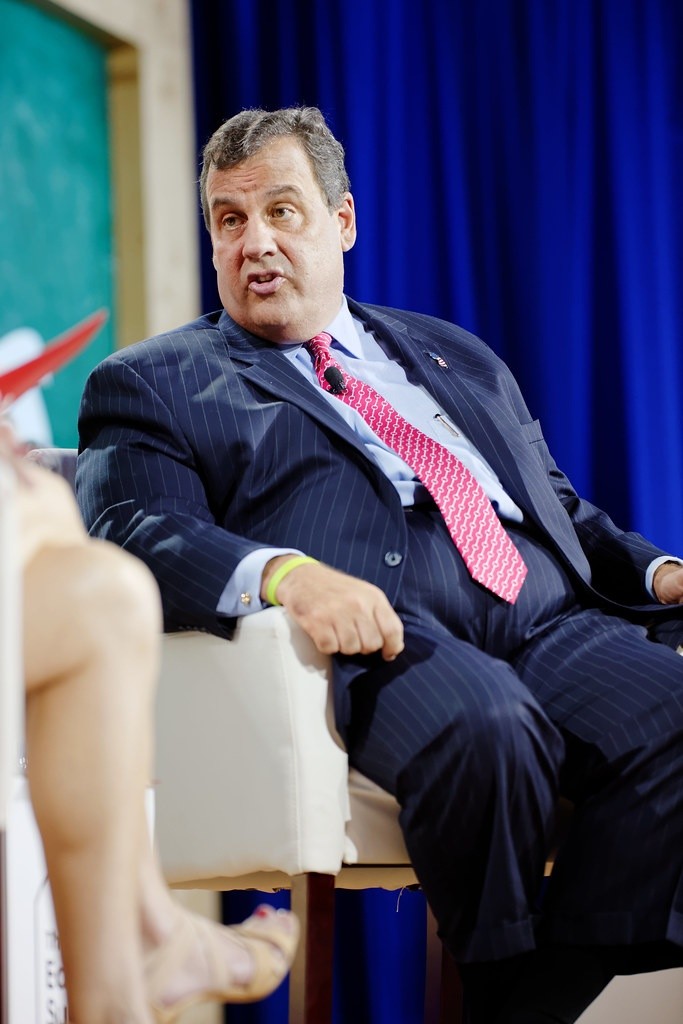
[76,108,682,1024]
[0,453,299,1024]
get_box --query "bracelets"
[265,555,318,607]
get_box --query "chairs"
[20,438,420,1024]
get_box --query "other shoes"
[460,962,610,1021]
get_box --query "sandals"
[142,903,300,1023]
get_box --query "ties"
[303,333,528,604]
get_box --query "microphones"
[324,367,347,394]
